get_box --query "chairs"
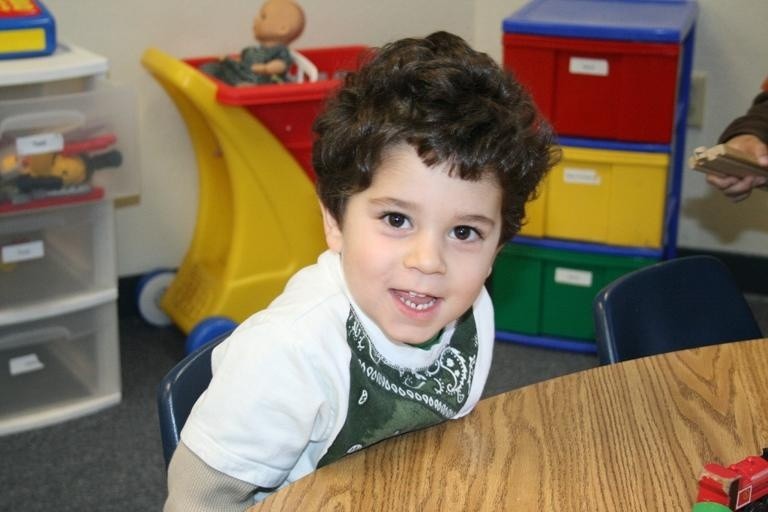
[592,255,765,365]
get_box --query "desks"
[241,333,767,511]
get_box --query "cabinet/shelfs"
[3,40,124,439]
[484,3,698,354]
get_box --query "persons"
[159,26,564,512]
[200,1,308,85]
[706,69,768,202]
